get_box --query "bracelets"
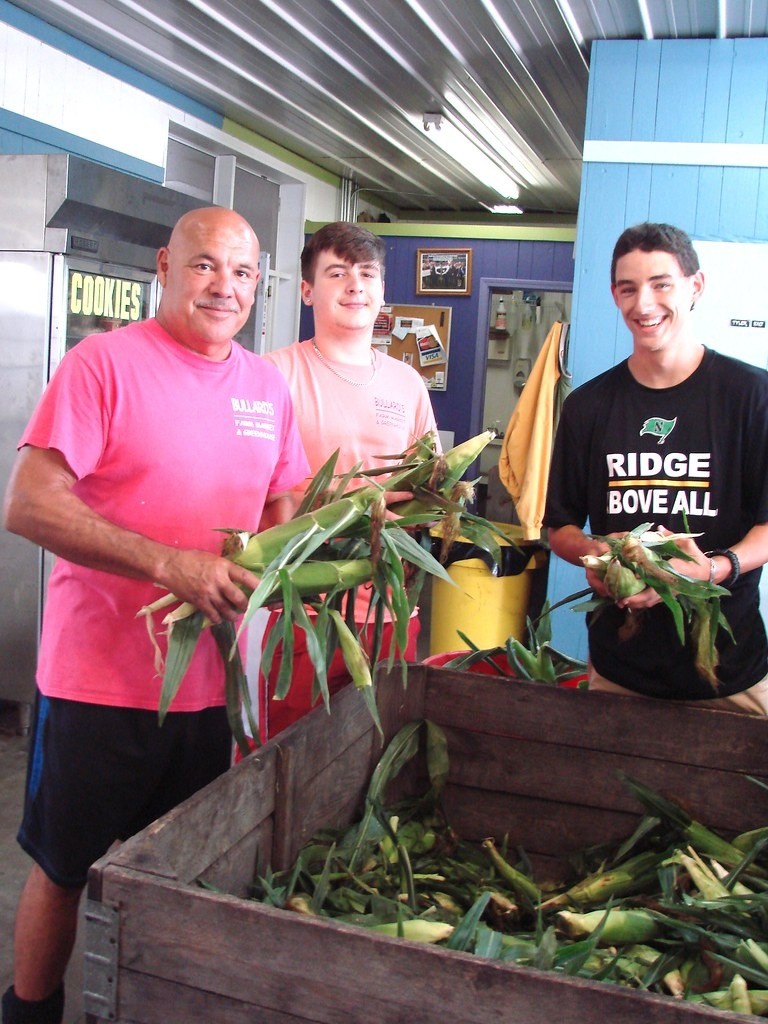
[705,548,739,589]
[708,556,717,584]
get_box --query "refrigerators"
[0,148,221,736]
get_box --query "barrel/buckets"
[421,649,592,692]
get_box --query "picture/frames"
[415,248,471,296]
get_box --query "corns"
[133,428,498,627]
[507,638,556,686]
[287,815,768,1021]
[582,531,668,599]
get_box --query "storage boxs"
[68,651,768,1022]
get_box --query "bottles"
[521,300,533,331]
[495,296,507,330]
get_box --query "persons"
[0,207,310,1024]
[232,221,444,773]
[543,221,768,717]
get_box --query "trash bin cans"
[428,519,548,657]
[421,650,589,689]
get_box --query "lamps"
[421,111,521,201]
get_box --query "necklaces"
[311,338,377,387]
